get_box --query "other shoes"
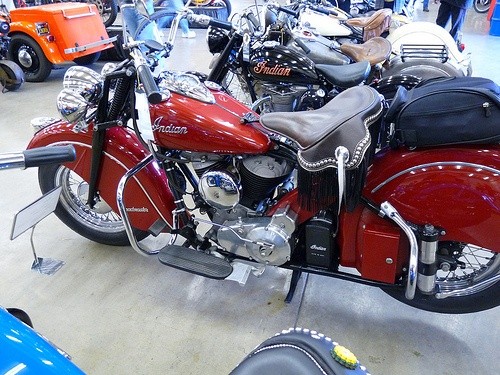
[423,7,429,12]
[158,29,164,37]
[181,30,197,38]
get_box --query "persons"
[327,0,350,14]
[436,0,473,42]
[423,0,429,12]
[375,0,405,15]
[118,0,163,92]
[156,0,196,37]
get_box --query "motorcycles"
[0,0,500,375]
